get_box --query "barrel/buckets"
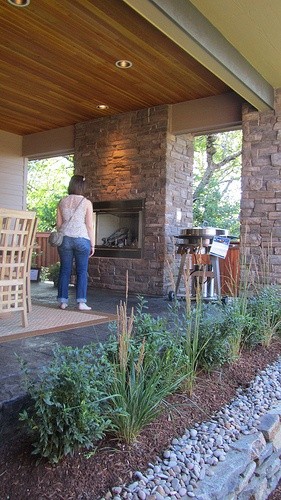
[192,264,215,297]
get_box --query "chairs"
[0,208,40,329]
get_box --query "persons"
[57,175,95,311]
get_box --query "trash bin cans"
[31,267,41,283]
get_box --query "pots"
[174,227,231,245]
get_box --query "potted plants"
[30,243,41,282]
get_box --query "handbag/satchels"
[48,196,86,246]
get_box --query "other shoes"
[61,302,92,311]
